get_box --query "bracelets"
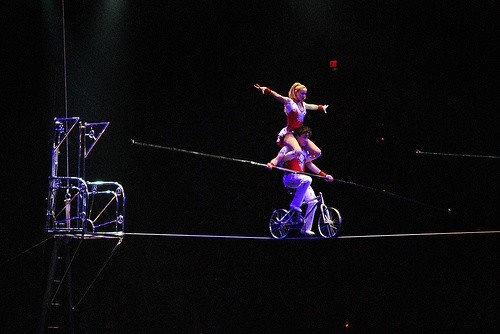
[265,87,271,93]
[318,104,322,110]
[319,171,327,177]
[270,162,274,167]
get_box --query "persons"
[254,82,328,170]
[266,126,334,236]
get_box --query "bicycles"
[267,177,343,239]
